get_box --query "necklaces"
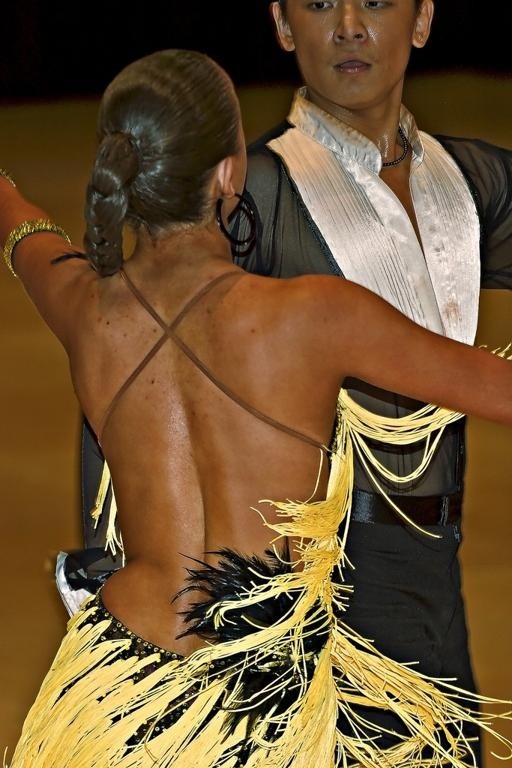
[384,125,409,166]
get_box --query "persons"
[0,48,512,768]
[77,1,511,768]
